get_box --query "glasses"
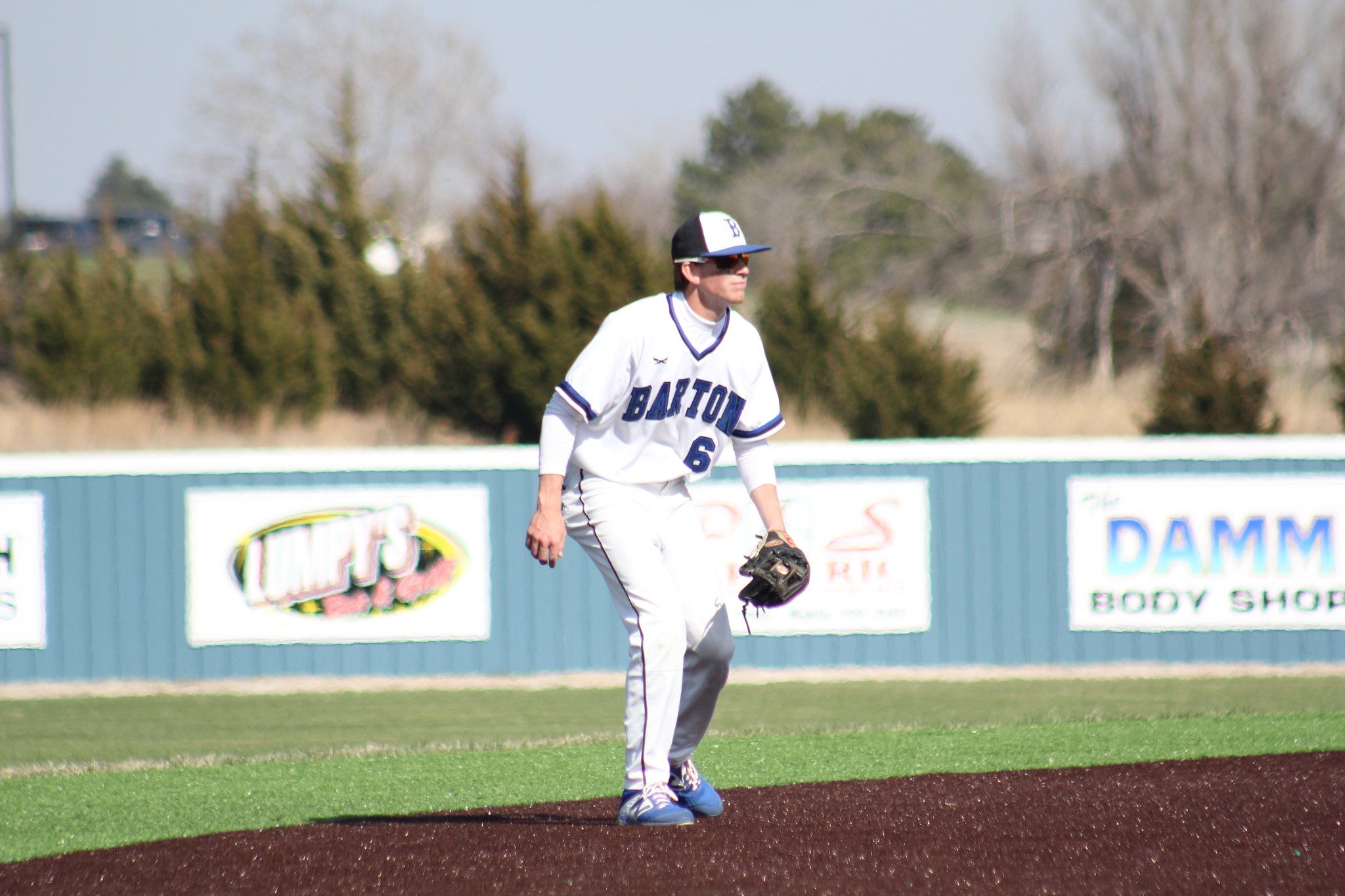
[673,253,750,269]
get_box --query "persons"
[527,211,810,828]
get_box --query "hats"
[671,210,772,260]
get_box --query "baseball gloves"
[737,528,810,637]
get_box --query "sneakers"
[617,782,695,826]
[669,758,724,817]
